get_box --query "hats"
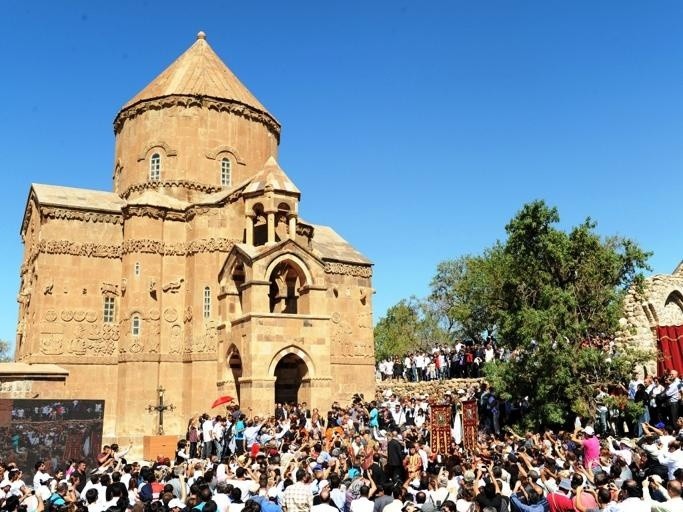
[558,478,574,491]
[619,437,634,448]
[462,470,476,482]
[139,483,154,499]
[580,425,596,436]
[655,422,666,430]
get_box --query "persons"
[369,330,618,384]
[1,369,683,512]
